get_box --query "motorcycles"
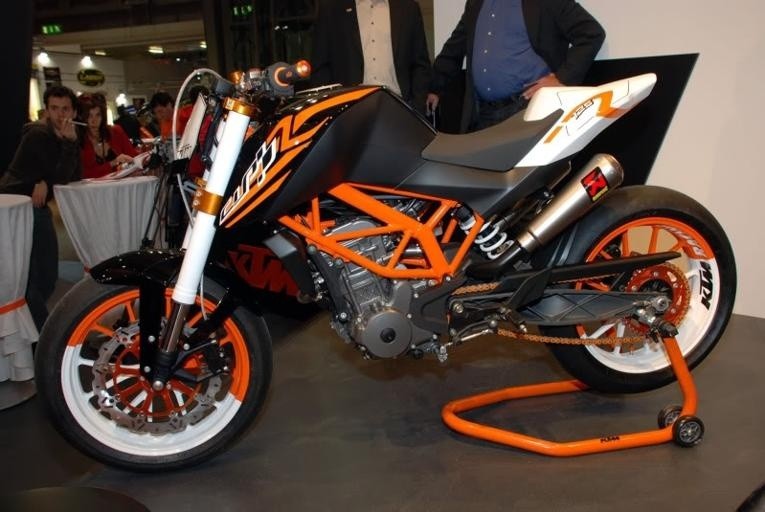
[32,53,736,472]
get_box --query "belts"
[478,93,520,109]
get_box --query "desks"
[2,176,169,411]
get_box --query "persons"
[311,1,431,124]
[1,85,209,356]
[424,0,607,135]
